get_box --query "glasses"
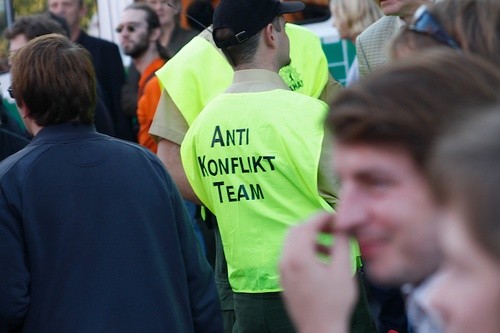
[406,3,465,52]
[116,26,134,31]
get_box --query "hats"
[211,0,306,46]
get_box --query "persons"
[330,0,500,86]
[148,24,346,333]
[180,0,376,333]
[415,106,499,333]
[47,1,137,144]
[278,54,500,333]
[116,0,190,155]
[0,33,224,333]
[4,14,114,136]
